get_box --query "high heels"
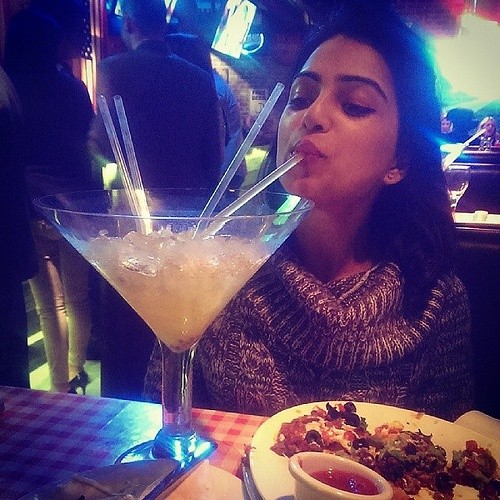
[67,371,88,395]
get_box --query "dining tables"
[0,386,272,500]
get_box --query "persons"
[0,3,248,394]
[440,116,461,142]
[154,12,479,423]
[473,116,500,145]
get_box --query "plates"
[248,400,500,500]
[454,213,500,227]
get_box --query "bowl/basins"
[287,452,393,500]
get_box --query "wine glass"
[33,189,315,483]
[444,166,470,223]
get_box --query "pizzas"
[269,402,500,500]
